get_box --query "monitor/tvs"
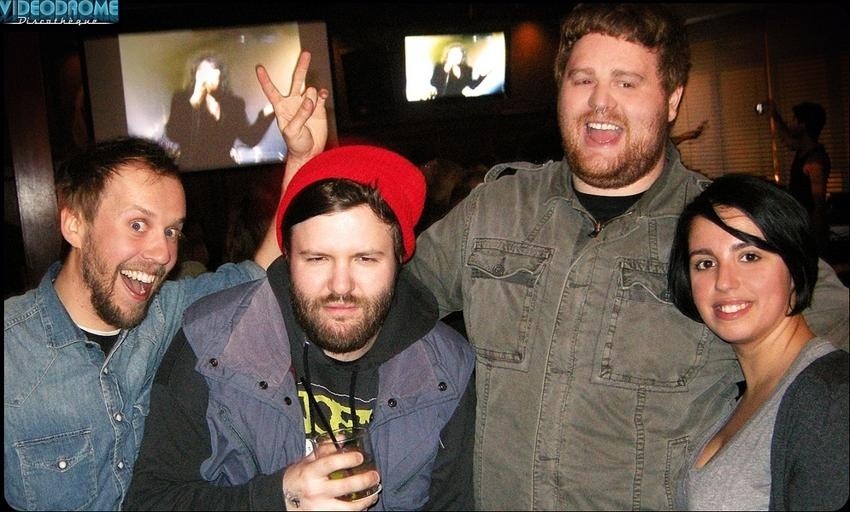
[79,12,342,177]
[398,28,511,107]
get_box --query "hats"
[276,146,428,263]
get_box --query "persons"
[165,50,276,173]
[666,173,849,511]
[431,43,487,99]
[402,0,849,511]
[4,49,329,511]
[760,98,831,262]
[122,143,476,511]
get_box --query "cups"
[311,427,383,501]
